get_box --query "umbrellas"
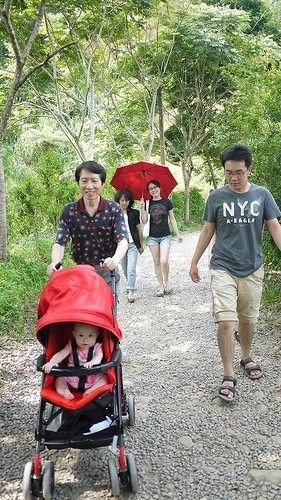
[109,161,177,206]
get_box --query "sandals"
[217,375,237,402]
[240,357,263,379]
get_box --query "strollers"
[23,258,140,500]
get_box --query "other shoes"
[158,286,172,296]
[126,289,135,303]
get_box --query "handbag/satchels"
[140,199,150,238]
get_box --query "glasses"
[224,170,245,177]
[149,187,159,192]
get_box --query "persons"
[189,144,281,401]
[113,189,144,303]
[46,161,129,293]
[140,180,183,297]
[42,323,109,400]
